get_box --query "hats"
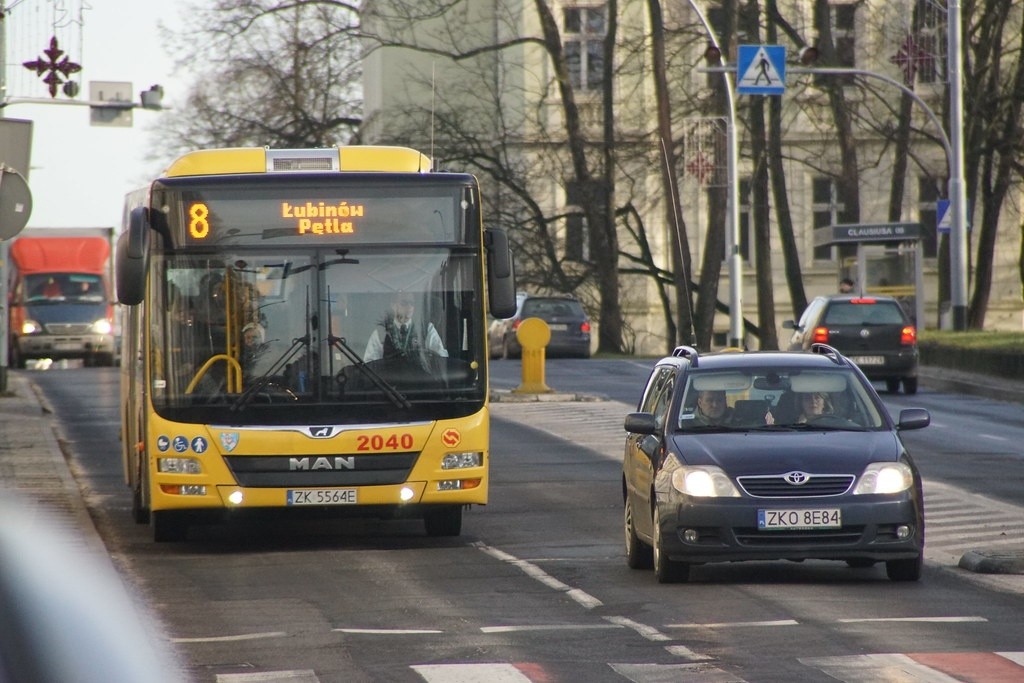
[242,322,265,343]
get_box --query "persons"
[837,280,852,295]
[764,391,852,426]
[164,272,449,403]
[687,389,747,428]
[78,282,91,296]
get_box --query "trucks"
[9,226,118,368]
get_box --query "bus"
[115,146,518,543]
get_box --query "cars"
[621,343,932,584]
[780,287,917,395]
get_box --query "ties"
[400,324,408,348]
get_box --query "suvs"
[486,290,591,359]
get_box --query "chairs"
[734,400,773,427]
[679,381,726,429]
